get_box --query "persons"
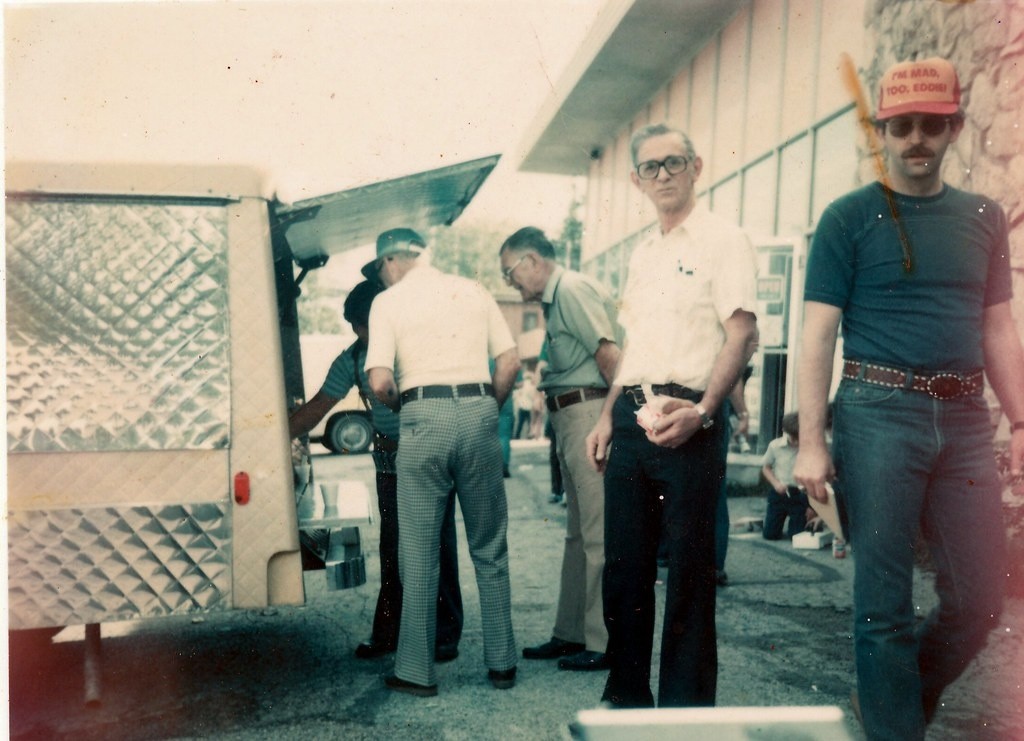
[498,227,622,672]
[657,361,749,585]
[292,281,464,661]
[762,413,815,539]
[585,125,759,707]
[796,58,1024,741]
[362,228,518,697]
[514,357,569,505]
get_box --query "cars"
[296,333,380,455]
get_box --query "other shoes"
[558,651,610,670]
[383,675,438,696]
[354,638,397,657]
[488,666,517,689]
[851,691,863,730]
[718,569,728,585]
[524,636,586,659]
[433,650,459,662]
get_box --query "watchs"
[694,403,713,431]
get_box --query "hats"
[360,227,427,281]
[876,58,961,120]
[343,280,387,328]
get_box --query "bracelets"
[736,412,749,419]
[1009,421,1024,434]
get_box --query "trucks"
[4,150,370,707]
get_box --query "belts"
[401,382,495,405]
[622,382,703,405]
[545,387,609,412]
[844,358,985,401]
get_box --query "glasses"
[502,255,528,287]
[637,155,691,180]
[886,116,951,139]
